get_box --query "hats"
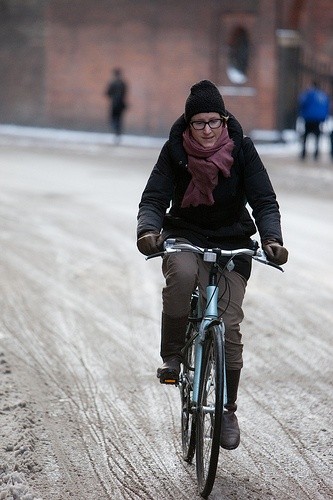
[185,79,226,123]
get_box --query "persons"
[296,78,329,162]
[107,67,127,146]
[137,80,288,450]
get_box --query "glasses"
[189,116,225,130]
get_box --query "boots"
[221,369,241,450]
[157,313,187,381]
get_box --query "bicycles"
[144,237,286,499]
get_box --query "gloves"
[136,234,166,256]
[263,240,289,265]
